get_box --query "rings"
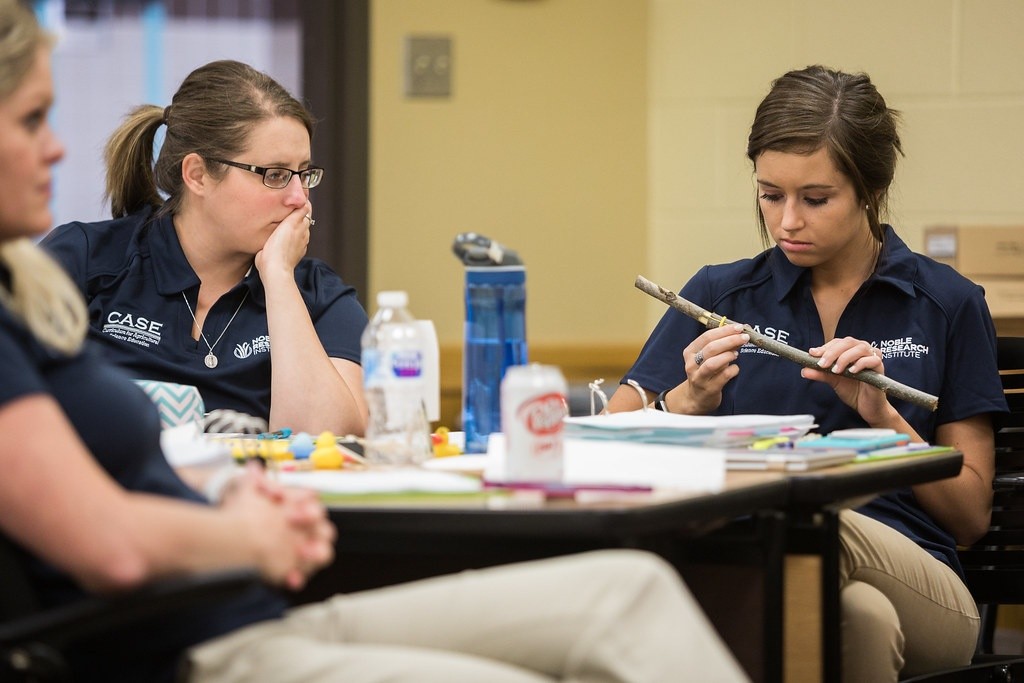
[305,214,316,226]
[694,350,706,365]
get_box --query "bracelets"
[654,389,671,412]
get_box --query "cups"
[450,234,529,455]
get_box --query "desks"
[270,433,963,683]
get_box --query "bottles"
[360,290,431,466]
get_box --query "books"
[726,446,860,472]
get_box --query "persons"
[1,0,754,683]
[598,65,1009,683]
[44,54,375,442]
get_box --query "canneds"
[498,362,568,484]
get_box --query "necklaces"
[176,283,249,368]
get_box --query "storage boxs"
[923,224,1024,279]
[972,279,1024,338]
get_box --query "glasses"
[206,157,324,189]
